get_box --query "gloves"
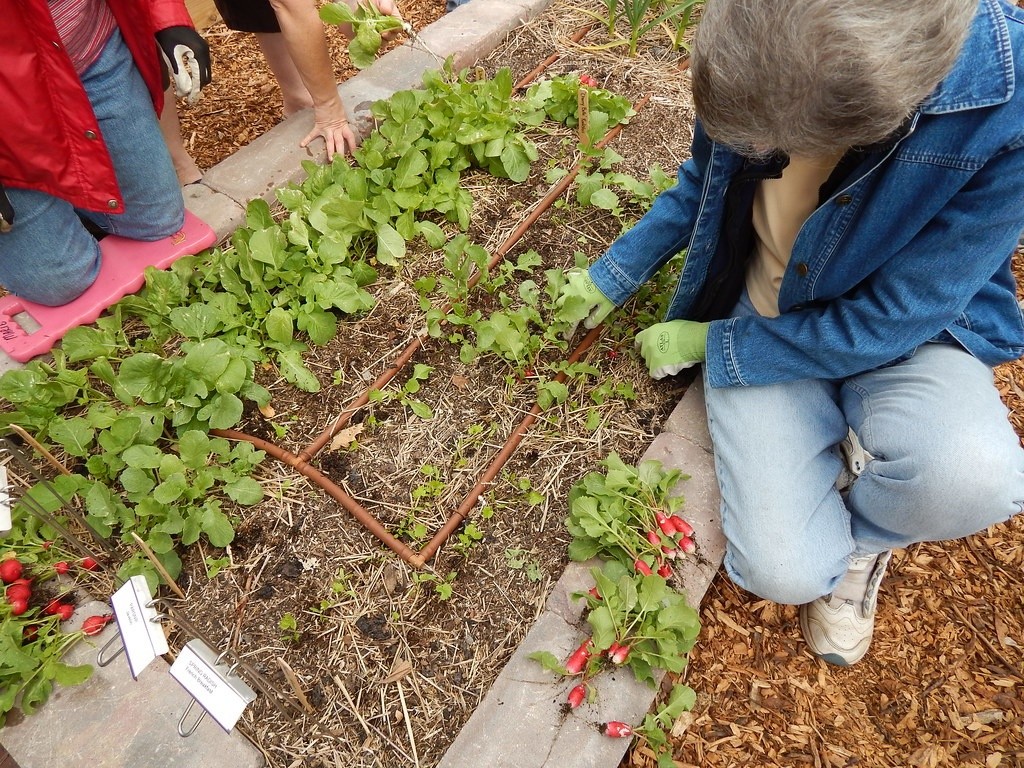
[636,320,711,380]
[556,268,616,340]
[155,25,213,105]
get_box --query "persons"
[556,0,1024,666]
[214,0,403,163]
[1,0,212,307]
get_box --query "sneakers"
[829,425,875,504]
[799,548,894,666]
